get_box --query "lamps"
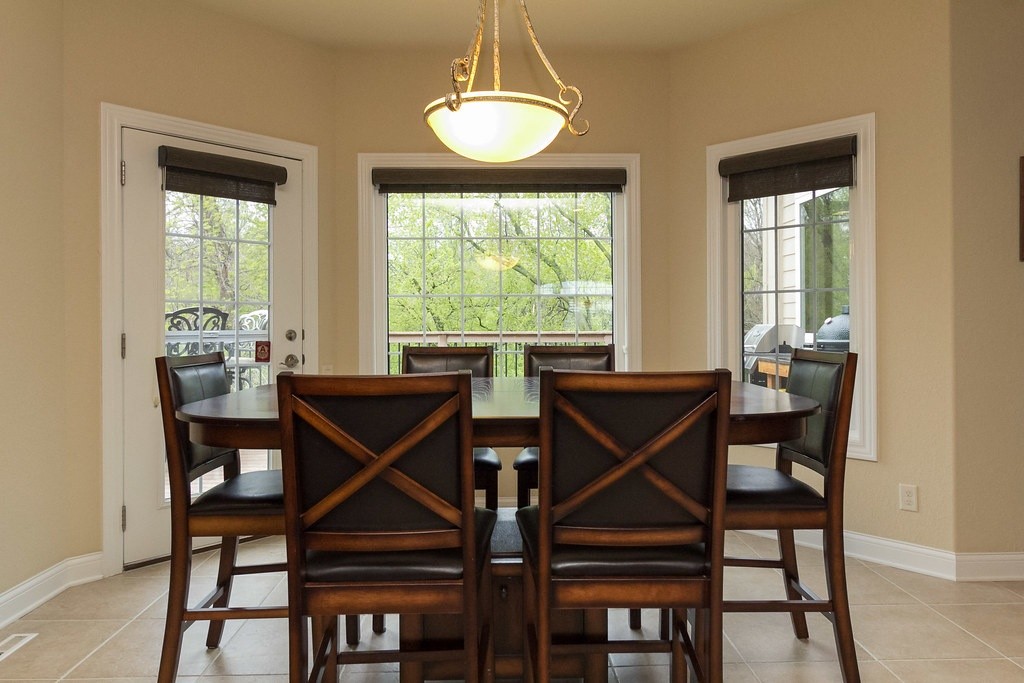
[424,1,589,162]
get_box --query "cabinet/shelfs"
[398,522,610,683]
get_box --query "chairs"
[400,344,503,511]
[511,343,616,507]
[276,371,499,683]
[630,346,862,683]
[163,307,269,390]
[154,352,361,682]
[515,366,731,683]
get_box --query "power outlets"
[900,483,919,511]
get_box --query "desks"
[174,377,822,683]
